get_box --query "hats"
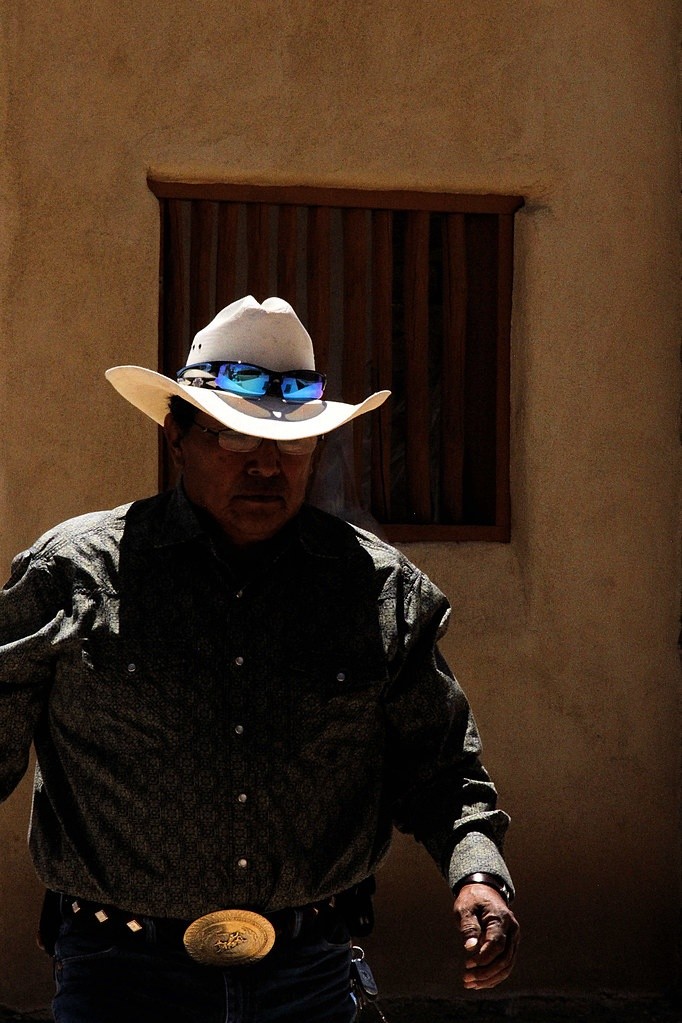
[103,295,392,440]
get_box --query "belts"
[61,875,378,968]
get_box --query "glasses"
[192,418,326,455]
[177,360,328,404]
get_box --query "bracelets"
[465,872,509,909]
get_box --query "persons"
[0,294,521,1023]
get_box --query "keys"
[349,959,388,1023]
